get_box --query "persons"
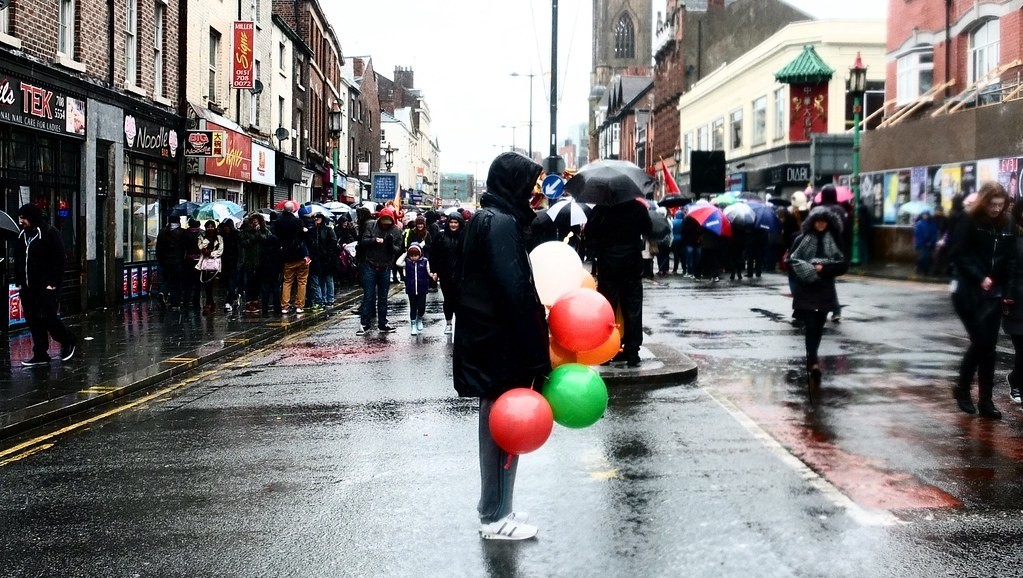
[951,181,1011,416]
[449,151,551,539]
[790,184,848,377]
[914,194,968,278]
[1006,172,1017,199]
[154,199,794,368]
[1001,197,1023,403]
[17,203,77,365]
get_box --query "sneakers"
[378,323,396,332]
[22,354,49,365]
[356,324,371,335]
[444,324,452,334]
[478,511,528,532]
[352,303,363,315]
[482,518,538,540]
[62,345,76,361]
[1006,374,1021,403]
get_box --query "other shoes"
[832,309,839,321]
[628,352,642,366]
[401,276,407,280]
[392,276,400,283]
[163,287,343,313]
[811,359,822,378]
[640,267,761,281]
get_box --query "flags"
[663,162,683,215]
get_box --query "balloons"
[489,241,625,455]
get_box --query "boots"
[411,316,417,335]
[417,316,424,330]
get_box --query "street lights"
[452,185,458,200]
[846,52,870,268]
[433,179,439,211]
[492,145,504,154]
[468,160,486,211]
[383,141,395,208]
[327,100,344,203]
[510,72,534,159]
[501,125,515,152]
[673,139,683,188]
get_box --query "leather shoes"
[953,385,975,414]
[977,399,1001,419]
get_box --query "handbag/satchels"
[201,251,220,270]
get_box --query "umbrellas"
[563,159,651,207]
[274,200,300,210]
[723,203,756,227]
[299,205,335,220]
[324,202,352,212]
[748,202,777,230]
[814,186,854,205]
[199,200,243,231]
[901,200,932,216]
[687,204,731,237]
[166,202,200,228]
[0,209,20,234]
[350,200,378,213]
